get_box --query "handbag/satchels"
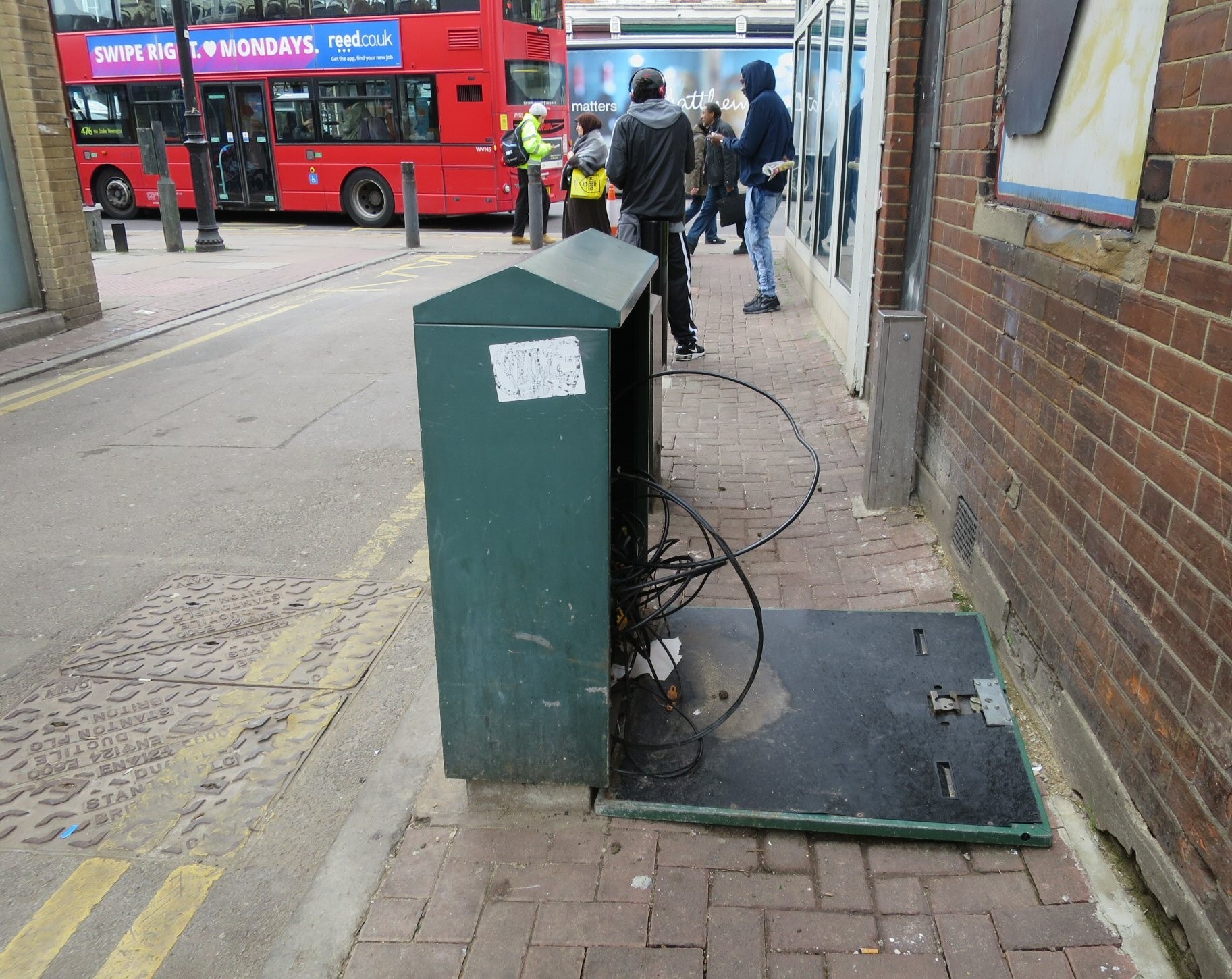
[718,190,746,228]
[569,168,607,199]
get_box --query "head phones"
[628,67,666,102]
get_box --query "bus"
[52,0,574,226]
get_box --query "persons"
[684,102,749,254]
[685,118,726,245]
[708,59,796,314]
[604,67,705,361]
[238,96,373,192]
[511,102,555,245]
[559,111,611,239]
[133,0,156,27]
[804,97,863,257]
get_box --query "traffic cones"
[607,184,620,238]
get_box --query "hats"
[631,68,663,89]
[529,103,547,116]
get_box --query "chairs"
[54,0,432,32]
[356,116,391,140]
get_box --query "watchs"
[720,136,725,148]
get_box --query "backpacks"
[501,119,536,167]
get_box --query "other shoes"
[706,236,725,244]
[734,247,747,253]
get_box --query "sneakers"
[743,294,780,314]
[544,234,556,244]
[744,289,762,306]
[676,343,704,361]
[512,235,530,245]
